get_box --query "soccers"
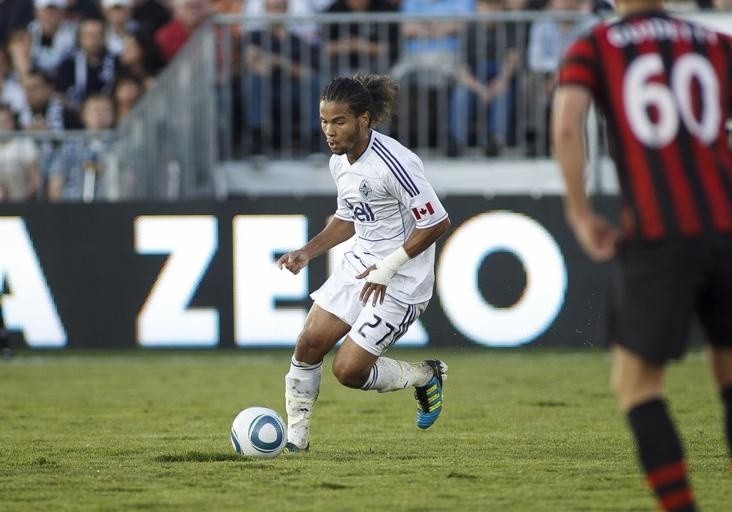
[231,407,287,459]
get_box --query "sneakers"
[282,441,310,452]
[414,358,448,430]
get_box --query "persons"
[546,0,732,512]
[274,70,452,452]
[1,1,732,203]
